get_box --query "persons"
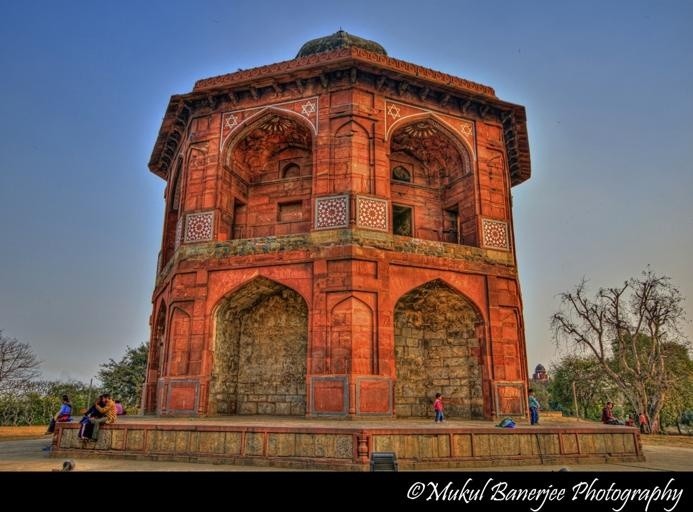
[629,413,636,427]
[528,389,540,425]
[45,395,73,435]
[639,413,646,434]
[433,393,446,424]
[79,394,126,442]
[602,402,625,425]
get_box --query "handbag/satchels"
[58,414,70,422]
